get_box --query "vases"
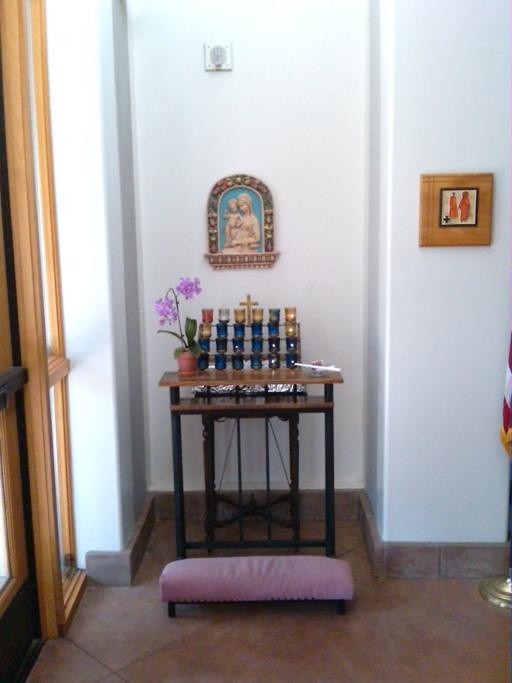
[178,352,198,377]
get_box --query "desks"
[157,367,344,559]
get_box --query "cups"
[196,306,298,369]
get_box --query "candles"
[195,307,298,370]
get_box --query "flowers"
[155,275,203,359]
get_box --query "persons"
[221,193,261,252]
[223,198,242,243]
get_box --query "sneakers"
[478,574,512,609]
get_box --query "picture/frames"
[205,173,281,271]
[416,173,494,247]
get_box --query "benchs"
[158,556,355,618]
